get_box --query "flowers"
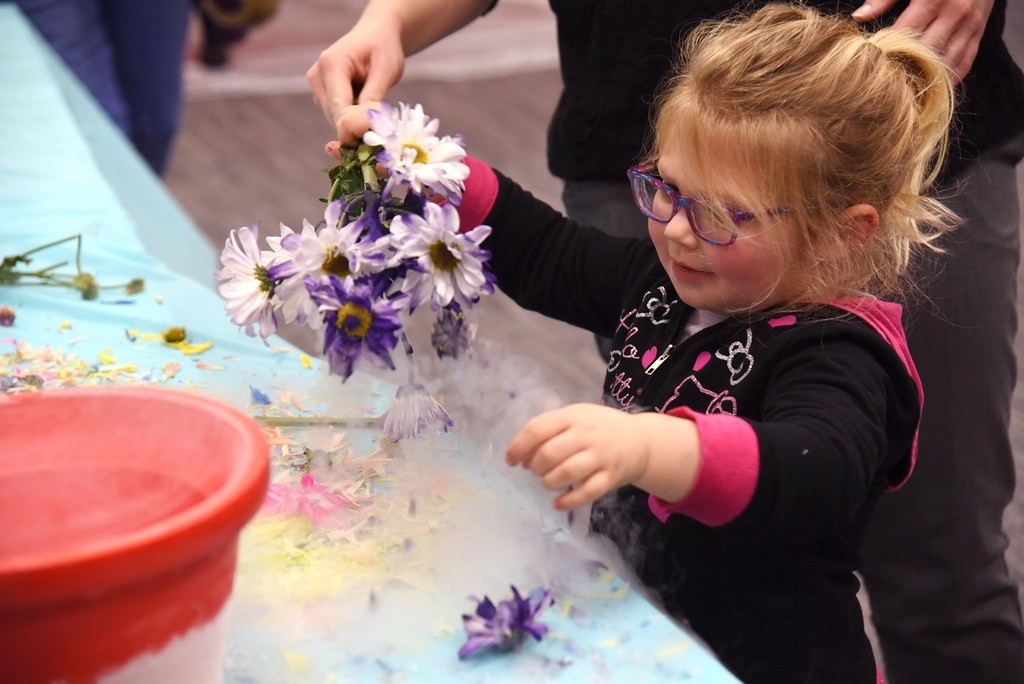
[0,98,626,667]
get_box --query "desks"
[0,3,748,683]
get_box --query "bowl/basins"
[2,384,271,684]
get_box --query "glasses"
[627,159,834,246]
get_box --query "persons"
[301,0,1024,684]
[323,4,965,684]
[0,1,280,187]
